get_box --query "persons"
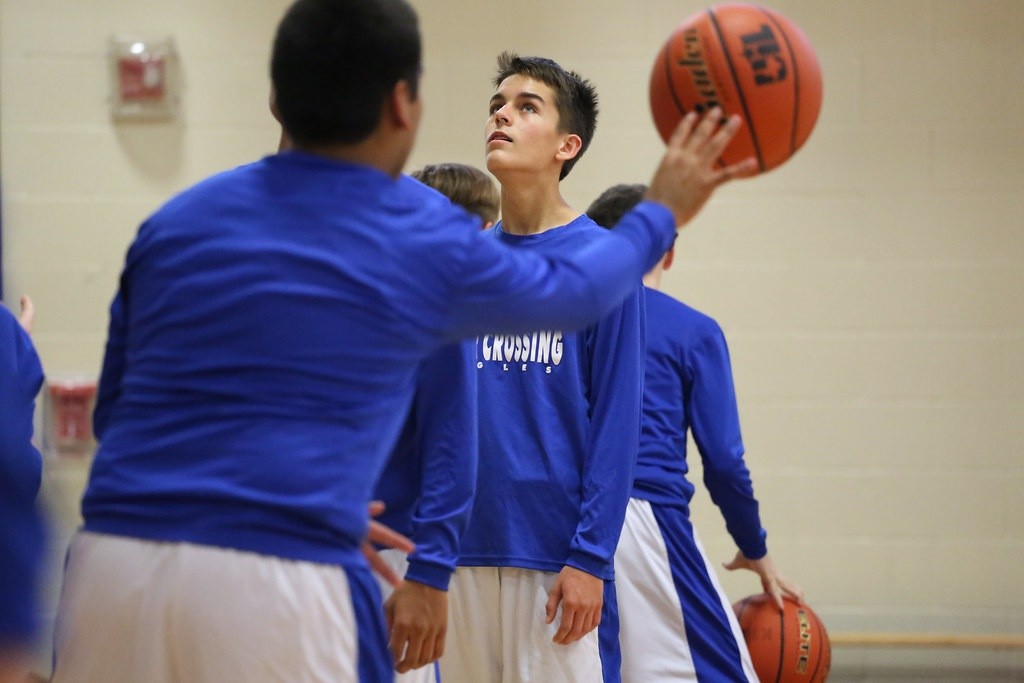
[0,0,806,683]
[53,0,758,683]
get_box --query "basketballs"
[722,586,841,683]
[646,0,825,184]
